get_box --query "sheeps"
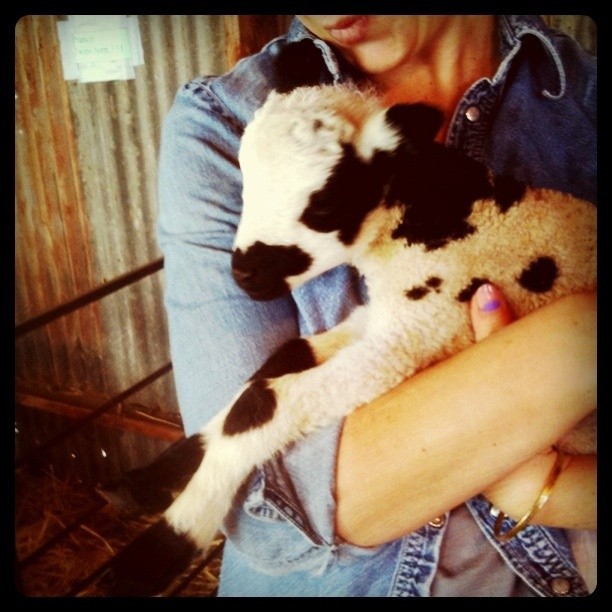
[67,82,598,604]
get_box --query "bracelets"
[493,449,566,542]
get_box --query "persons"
[156,16,598,595]
